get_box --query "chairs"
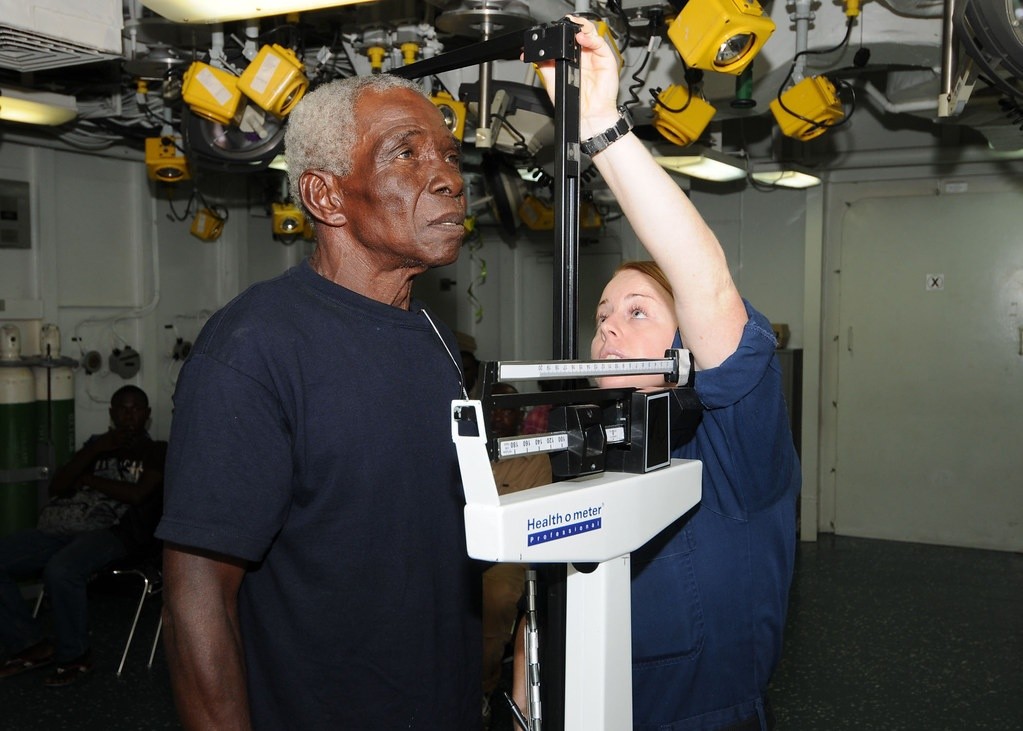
[29,565,162,679]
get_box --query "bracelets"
[581,106,632,156]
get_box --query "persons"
[513,19,801,730]
[460,351,552,730]
[0,386,166,685]
[153,75,484,731]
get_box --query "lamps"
[272,202,304,235]
[517,196,602,231]
[190,211,224,241]
[181,61,248,125]
[145,137,192,183]
[769,76,844,142]
[236,43,310,117]
[652,85,717,148]
[666,0,776,76]
[429,88,466,143]
